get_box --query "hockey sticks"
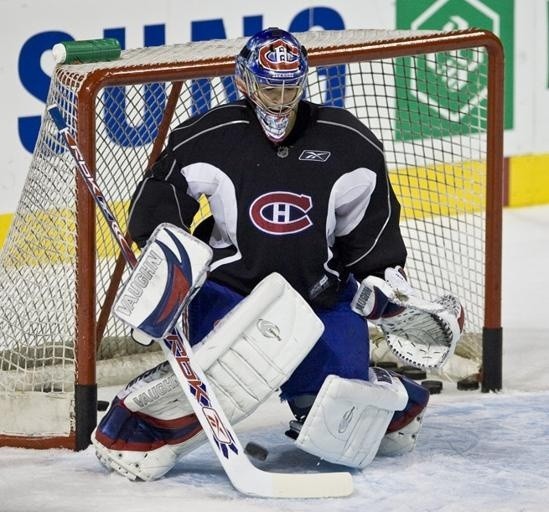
[48,104,355,498]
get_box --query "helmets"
[235,27,308,142]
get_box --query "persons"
[86,26,429,484]
